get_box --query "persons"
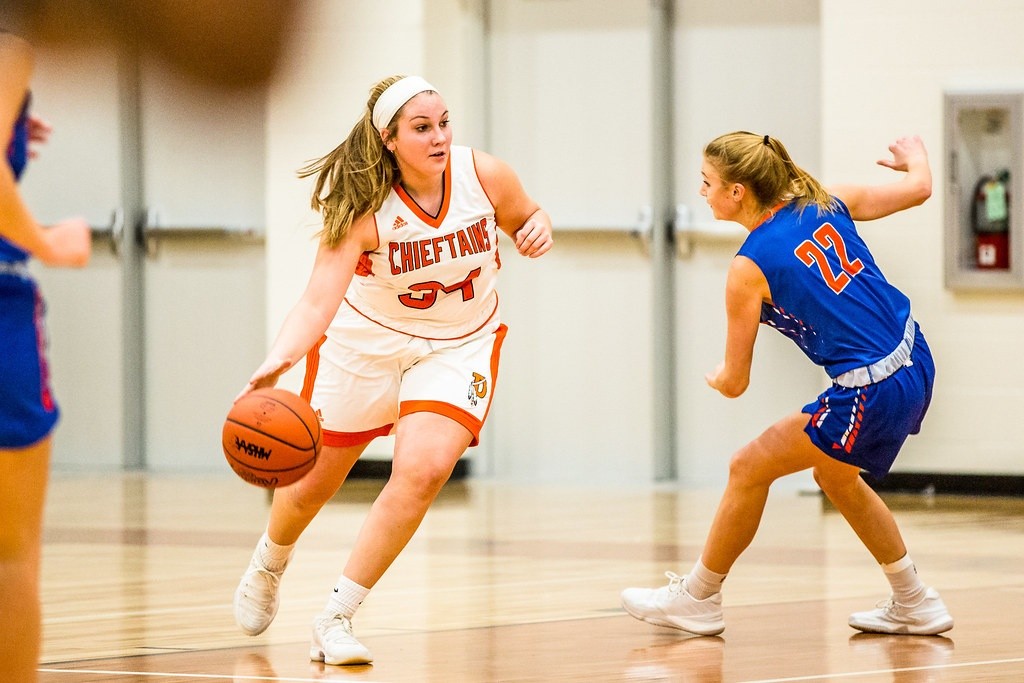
[614,132,955,639]
[234,72,551,667]
[1,23,87,677]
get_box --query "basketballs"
[223,388,324,487]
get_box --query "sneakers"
[232,533,297,635]
[620,575,727,635]
[847,588,957,634]
[309,615,374,665]
